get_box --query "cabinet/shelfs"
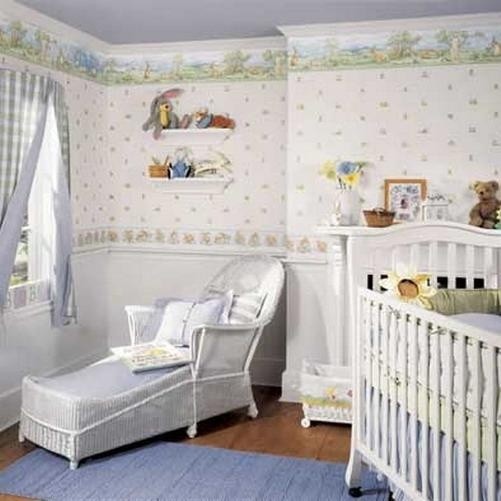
[144,127,234,194]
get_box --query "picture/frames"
[382,178,428,224]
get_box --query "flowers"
[317,159,368,191]
[375,262,439,313]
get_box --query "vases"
[332,188,365,226]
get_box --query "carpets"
[0,438,392,501]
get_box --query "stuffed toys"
[167,147,194,178]
[468,180,501,229]
[142,88,181,140]
[193,109,235,128]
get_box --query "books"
[109,336,188,374]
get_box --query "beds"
[346,220,500,501]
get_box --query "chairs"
[17,252,287,473]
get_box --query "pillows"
[200,285,268,325]
[138,288,237,348]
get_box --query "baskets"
[364,208,394,227]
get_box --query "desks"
[314,224,500,365]
